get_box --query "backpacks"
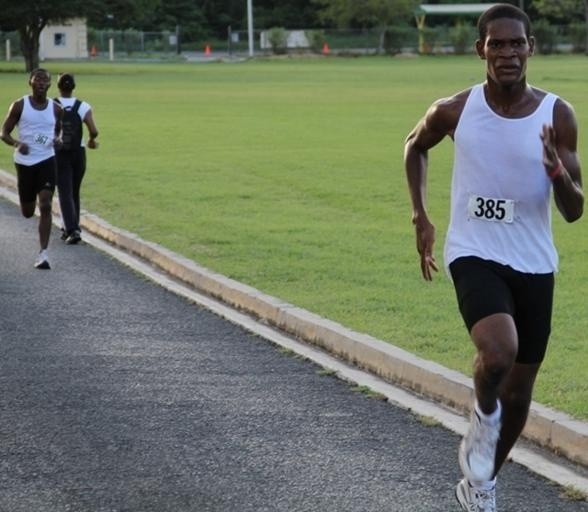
[53,98,82,152]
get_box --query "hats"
[57,73,75,88]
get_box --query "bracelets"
[548,158,562,178]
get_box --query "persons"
[0,67,98,270]
[403,4,585,512]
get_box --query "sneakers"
[34,250,51,269]
[455,407,502,512]
[60,227,81,244]
